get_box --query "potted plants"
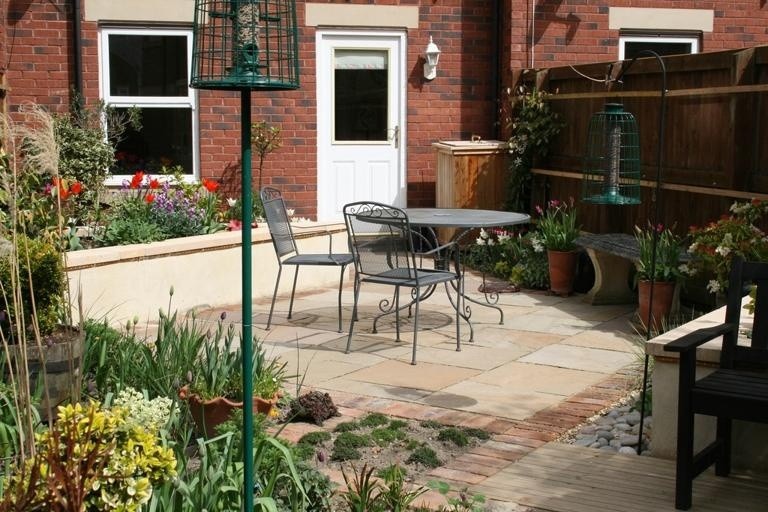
[178,310,286,402]
[0,232,86,425]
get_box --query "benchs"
[573,232,696,313]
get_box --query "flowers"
[633,222,684,281]
[537,196,582,252]
[686,195,767,293]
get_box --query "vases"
[181,386,281,441]
[714,291,727,309]
[637,279,672,330]
[545,247,573,298]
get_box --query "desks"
[356,209,532,352]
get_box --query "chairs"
[343,201,462,366]
[661,256,767,512]
[262,185,362,334]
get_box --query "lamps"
[421,34,441,81]
